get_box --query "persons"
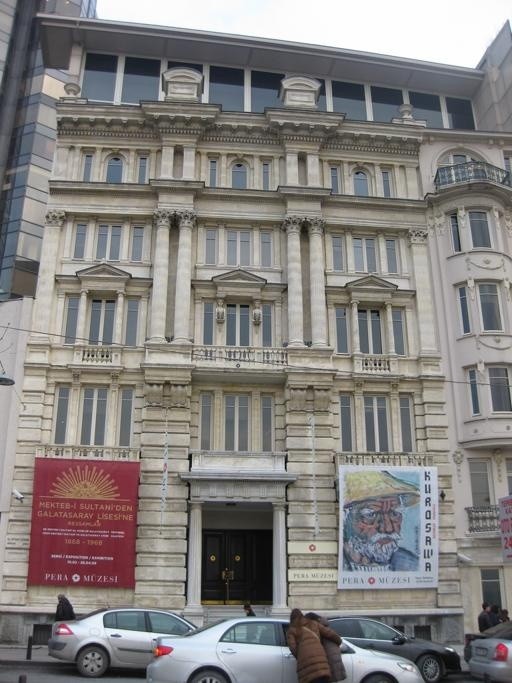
[343,471,419,575]
[305,612,346,682]
[478,600,509,632]
[55,594,75,620]
[243,603,256,641]
[286,608,342,683]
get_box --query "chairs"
[245,627,273,643]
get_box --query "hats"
[344,471,421,507]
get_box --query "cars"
[47,606,199,677]
[145,615,427,683]
[465,620,512,662]
[258,615,463,682]
[467,626,512,682]
